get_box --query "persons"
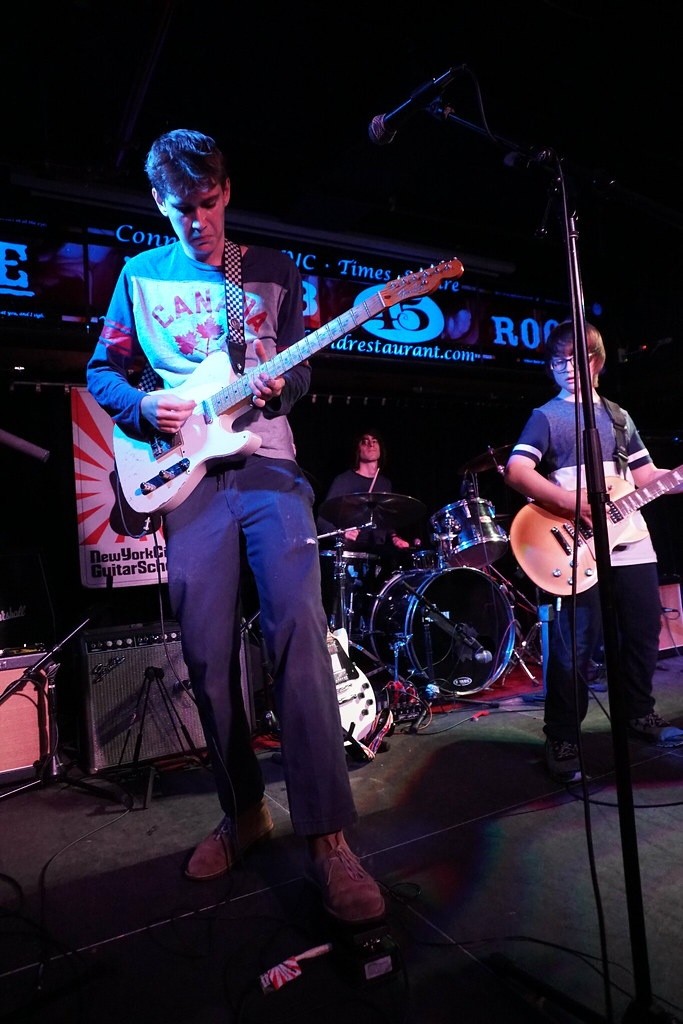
[504,322,683,774]
[87,129,386,923]
[317,427,410,554]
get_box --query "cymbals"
[319,491,429,525]
[457,443,517,474]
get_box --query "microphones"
[174,679,192,692]
[474,650,493,664]
[460,469,470,497]
[367,65,461,148]
[662,607,678,612]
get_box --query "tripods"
[318,508,499,734]
[0,618,129,804]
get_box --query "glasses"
[548,351,597,371]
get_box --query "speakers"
[70,616,257,779]
[656,572,683,659]
[0,645,54,785]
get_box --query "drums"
[374,549,437,587]
[427,496,508,569]
[319,549,381,643]
[368,567,517,695]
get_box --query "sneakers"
[303,830,385,922]
[185,802,276,881]
[629,711,683,747]
[544,734,582,780]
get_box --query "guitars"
[506,463,683,597]
[111,256,464,515]
